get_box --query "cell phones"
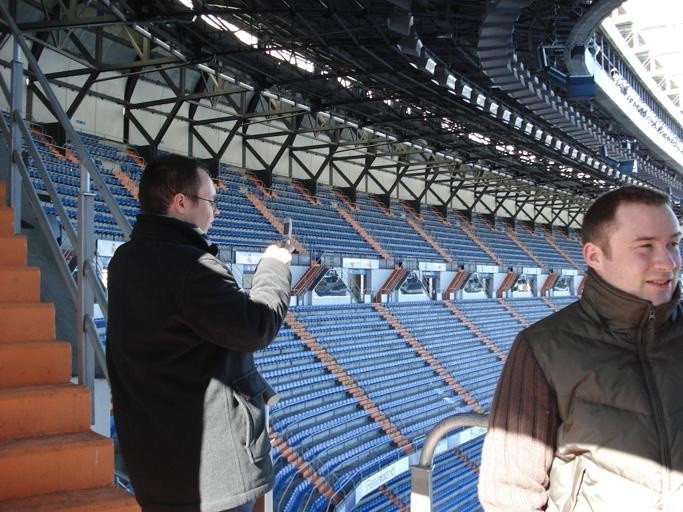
[280,217,292,248]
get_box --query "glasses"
[185,192,219,210]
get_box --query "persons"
[105,153,295,512]
[477,185,683,512]
